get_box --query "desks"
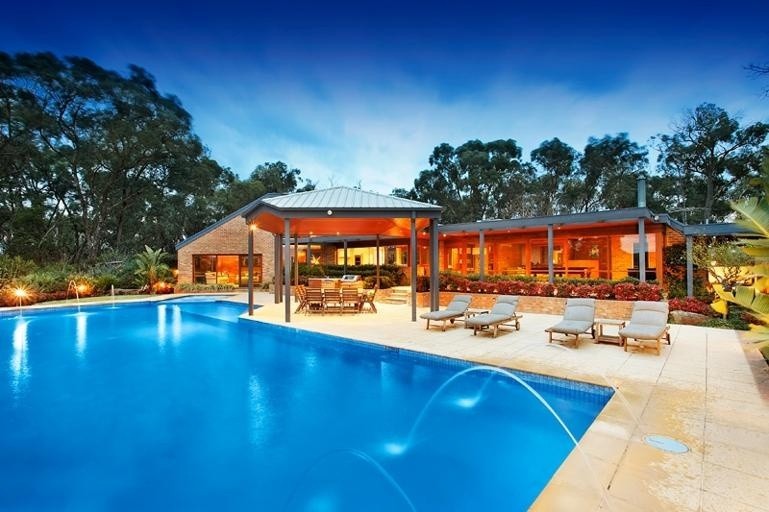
[531,266,594,278]
[627,266,656,279]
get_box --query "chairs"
[419,293,525,338]
[293,274,379,317]
[546,298,672,355]
